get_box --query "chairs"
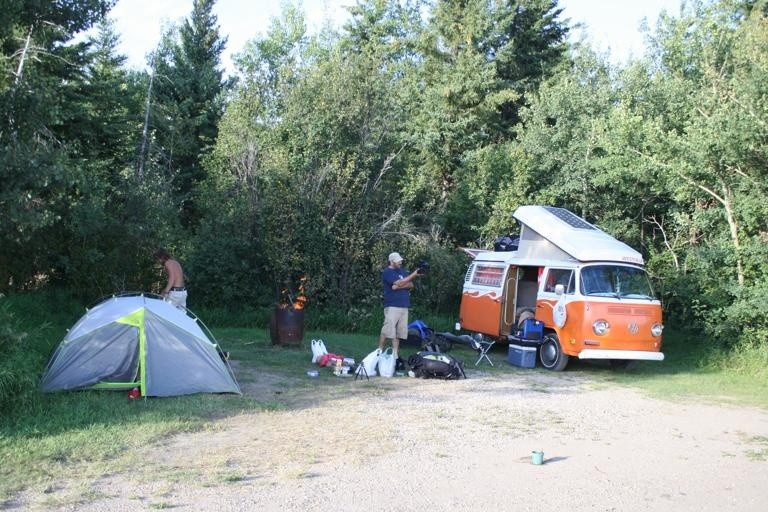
[517,279,537,315]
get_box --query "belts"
[170,286,187,292]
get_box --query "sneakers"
[395,358,405,369]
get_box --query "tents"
[38,290,243,395]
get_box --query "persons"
[154,248,188,315]
[376,252,424,371]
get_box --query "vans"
[455,205,664,372]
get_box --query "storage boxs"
[508,343,537,369]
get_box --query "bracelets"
[164,292,168,294]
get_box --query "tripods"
[355,362,370,382]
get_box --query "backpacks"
[409,351,466,380]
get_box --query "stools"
[470,337,496,368]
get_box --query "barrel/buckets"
[276,308,303,344]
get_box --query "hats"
[388,252,403,263]
